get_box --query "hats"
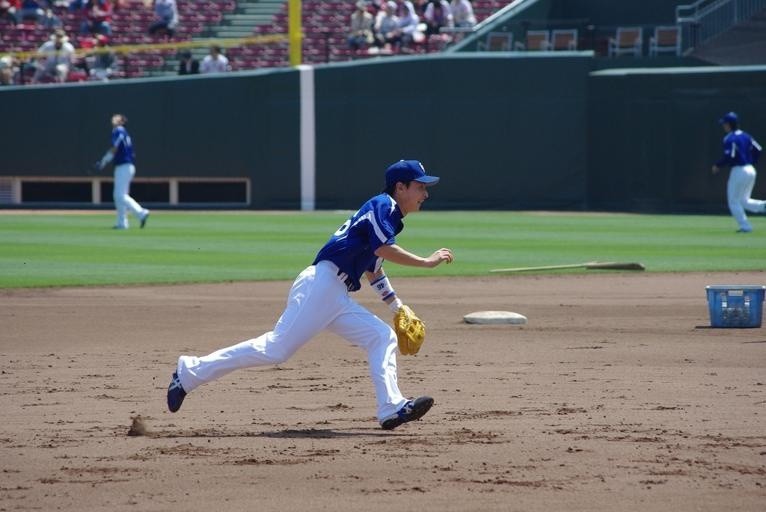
[385,160,441,188]
[719,112,738,124]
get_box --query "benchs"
[1,0,512,83]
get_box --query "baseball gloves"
[394,304,425,356]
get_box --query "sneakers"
[111,209,150,229]
[168,368,187,412]
[736,228,752,232]
[381,396,434,430]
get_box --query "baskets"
[706,284,766,328]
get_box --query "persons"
[343,0,479,56]
[176,48,199,76]
[0,0,179,85]
[165,159,453,430]
[711,110,765,232]
[98,114,149,230]
[197,44,229,73]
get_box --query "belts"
[337,270,355,292]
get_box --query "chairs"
[606,25,683,58]
[514,28,579,53]
